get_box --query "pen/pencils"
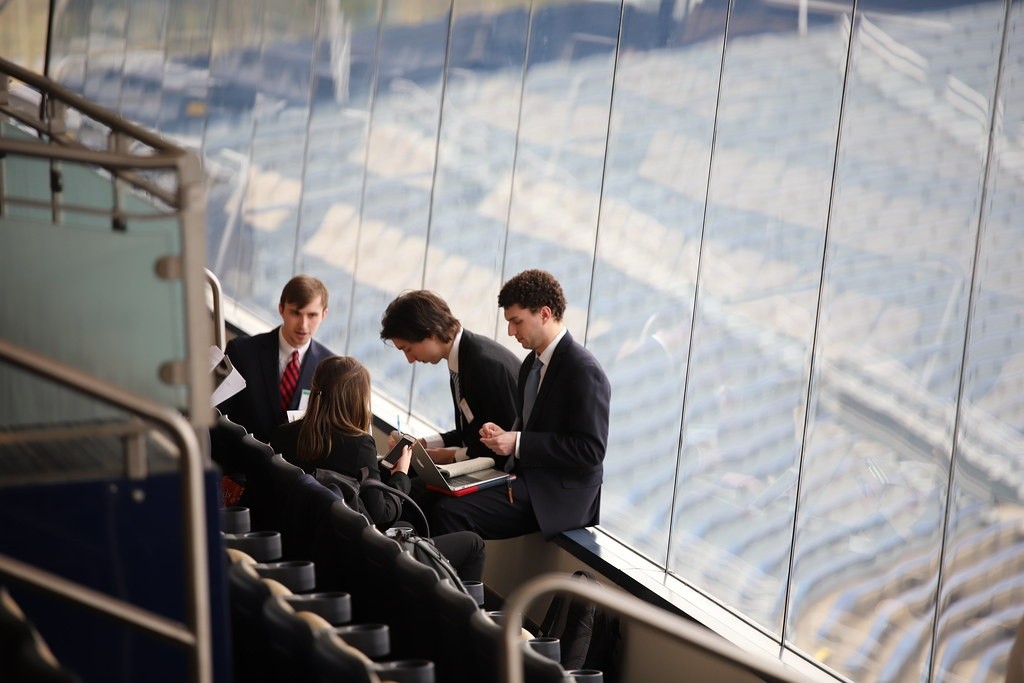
[397,414,400,436]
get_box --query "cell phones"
[380,434,417,469]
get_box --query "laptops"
[403,439,510,492]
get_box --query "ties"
[449,368,462,434]
[522,358,544,432]
[279,351,302,425]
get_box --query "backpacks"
[536,570,606,671]
[314,467,430,543]
[384,528,472,598]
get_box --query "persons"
[273,357,487,579]
[381,288,522,463]
[219,276,338,447]
[418,269,614,539]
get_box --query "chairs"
[0,401,603,683]
